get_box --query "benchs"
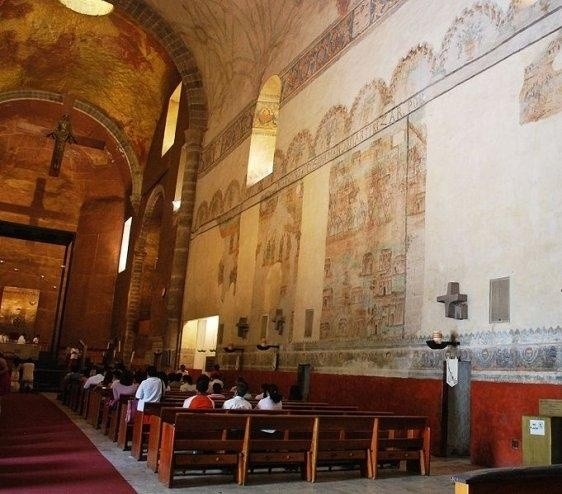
[63,372,431,489]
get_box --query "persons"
[65,365,282,434]
[64,343,79,368]
[0,329,40,344]
[0,352,34,393]
